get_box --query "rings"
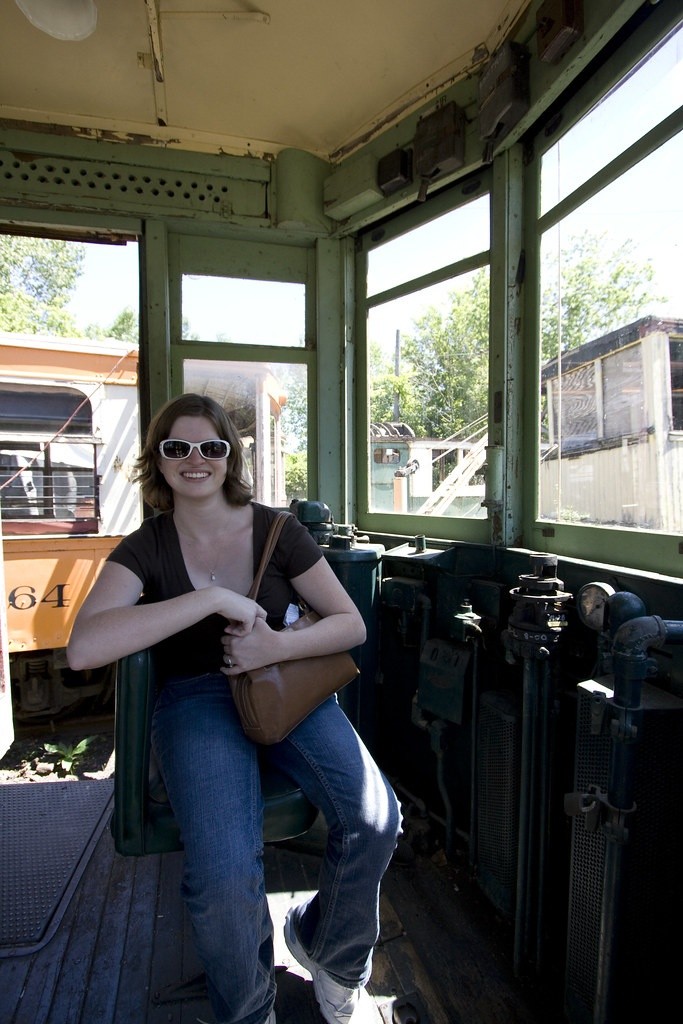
[229,658,232,667]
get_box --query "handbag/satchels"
[230,510,362,745]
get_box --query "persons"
[65,393,404,1024]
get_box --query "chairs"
[112,642,321,1004]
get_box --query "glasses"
[158,439,231,461]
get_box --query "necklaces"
[204,562,220,582]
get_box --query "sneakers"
[284,908,382,1024]
